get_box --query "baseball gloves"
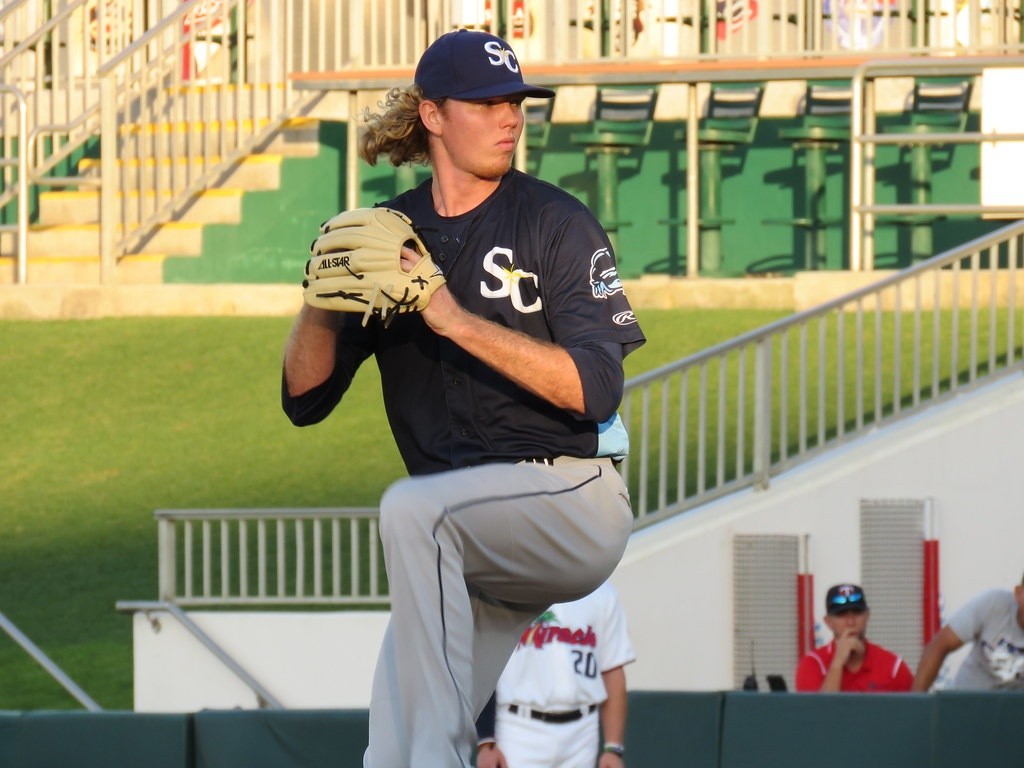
[300,204,448,331]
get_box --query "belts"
[508,704,596,724]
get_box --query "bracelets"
[604,744,626,756]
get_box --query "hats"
[414,28,556,100]
[826,584,866,614]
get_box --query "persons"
[464,584,635,768]
[280,31,647,767]
[791,584,917,692]
[911,574,1024,692]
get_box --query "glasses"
[831,593,862,604]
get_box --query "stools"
[523,77,976,279]
